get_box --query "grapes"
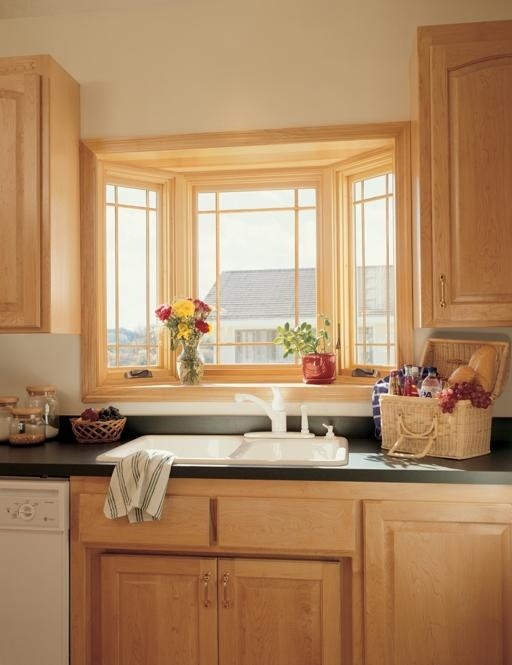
[438,382,491,414]
[99,406,124,420]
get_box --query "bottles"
[389,364,442,399]
[0,385,59,443]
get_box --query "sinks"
[229,433,348,467]
[95,433,245,463]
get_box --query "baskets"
[379,338,510,460]
[69,416,127,444]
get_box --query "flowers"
[155,297,212,346]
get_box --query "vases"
[176,339,205,385]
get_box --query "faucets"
[234,385,287,432]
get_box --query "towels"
[103,448,175,524]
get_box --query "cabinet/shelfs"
[361,483,512,665]
[410,20,512,330]
[0,55,82,336]
[69,475,362,665]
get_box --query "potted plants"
[272,310,336,383]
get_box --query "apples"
[81,408,99,421]
